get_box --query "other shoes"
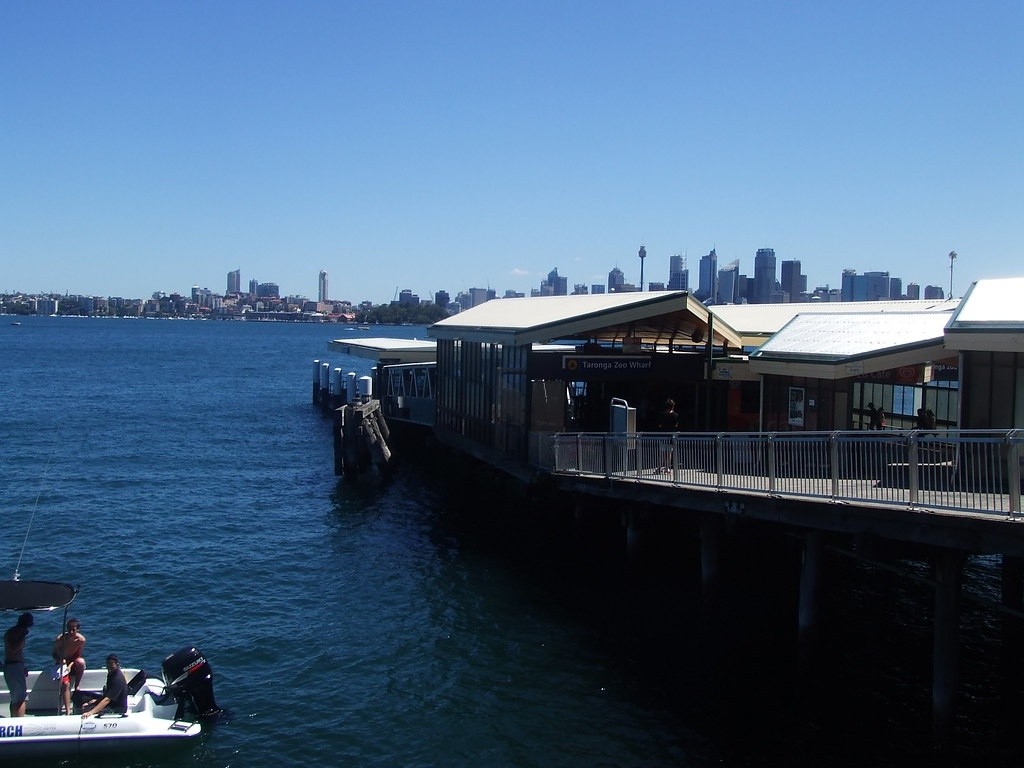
[664,469,671,475]
[653,468,663,474]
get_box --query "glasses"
[23,622,34,626]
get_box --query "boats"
[1,572,212,758]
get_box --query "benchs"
[852,419,940,440]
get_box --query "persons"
[867,401,936,439]
[81,653,127,719]
[651,399,679,478]
[51,616,86,714]
[4,612,33,718]
